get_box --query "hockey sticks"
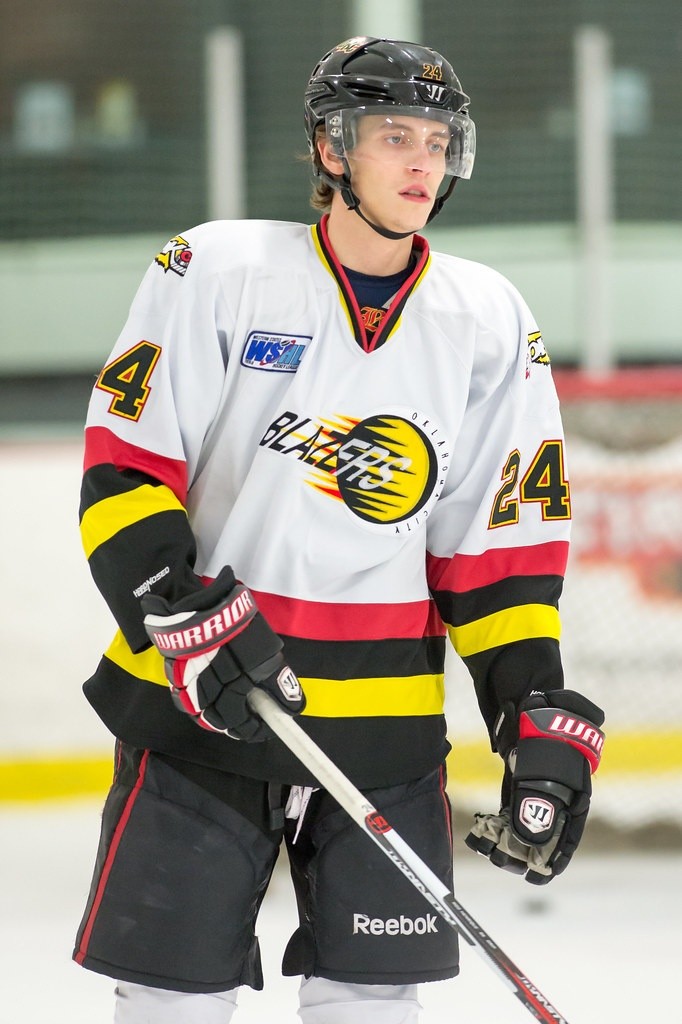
[244,685,568,1024]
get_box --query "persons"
[72,35,606,1024]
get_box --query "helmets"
[305,36,473,159]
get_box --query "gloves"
[464,690,606,886]
[141,565,307,743]
